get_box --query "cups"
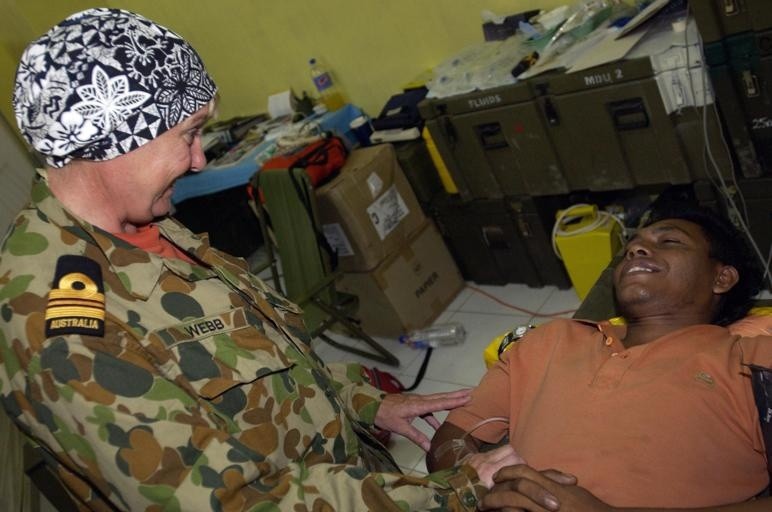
[312,105,327,115]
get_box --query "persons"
[0,7,526,512]
[426,204,772,512]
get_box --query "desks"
[172,105,368,206]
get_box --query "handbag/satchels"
[248,136,348,204]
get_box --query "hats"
[11,8,218,167]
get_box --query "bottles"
[398,323,466,349]
[308,57,344,112]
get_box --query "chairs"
[249,168,401,367]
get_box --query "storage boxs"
[250,104,465,339]
[385,2,772,291]
